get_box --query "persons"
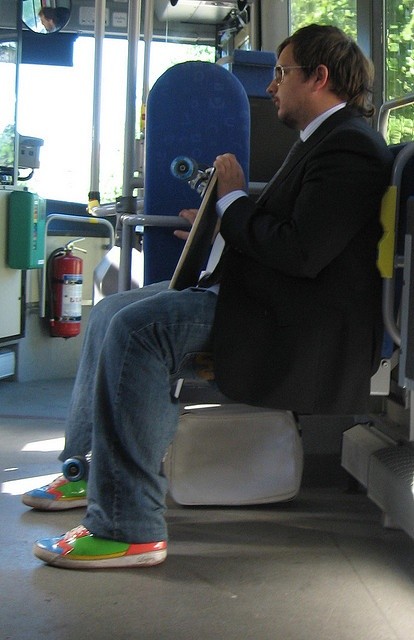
[46,7,70,32]
[21,23,395,569]
[38,7,56,34]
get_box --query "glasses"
[273,66,304,86]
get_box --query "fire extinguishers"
[46,236,87,338]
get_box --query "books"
[167,169,219,291]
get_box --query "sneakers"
[21,476,89,510]
[32,526,166,570]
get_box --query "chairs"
[245,92,298,185]
[167,131,412,413]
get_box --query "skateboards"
[63,157,220,481]
